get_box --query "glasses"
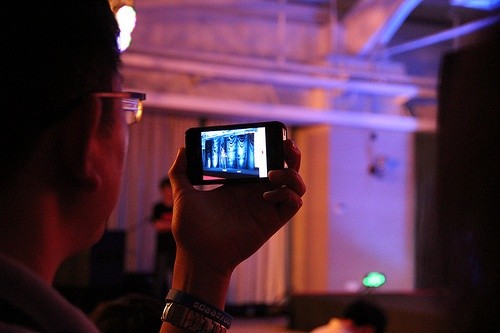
[94,92,145,125]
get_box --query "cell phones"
[184,121,288,186]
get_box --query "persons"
[308,299,388,333]
[0,0,307,333]
[150,178,177,289]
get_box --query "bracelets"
[165,289,233,330]
[161,302,228,333]
